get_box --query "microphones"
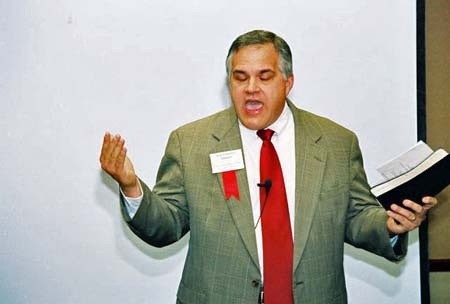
[265,180,271,192]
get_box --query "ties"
[256,128,294,304]
